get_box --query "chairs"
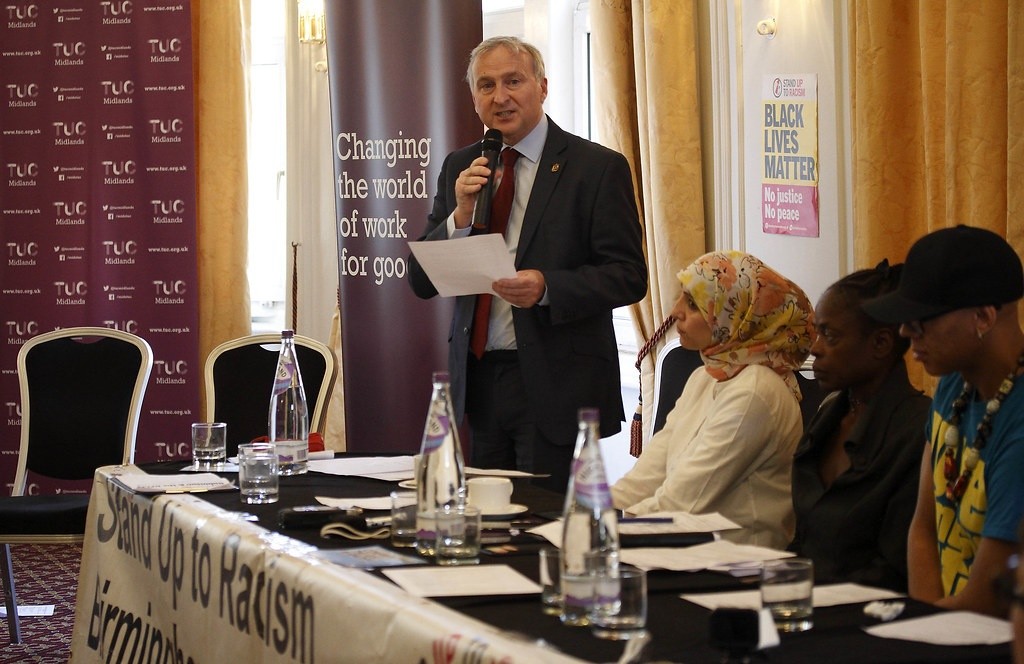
[204,333,337,458]
[650,339,821,451]
[0,327,155,644]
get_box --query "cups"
[238,443,276,455]
[389,491,419,548]
[539,549,565,615]
[758,559,814,632]
[414,455,421,479]
[239,453,279,503]
[434,504,481,567]
[192,422,227,466]
[590,568,648,641]
[468,477,512,512]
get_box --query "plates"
[464,503,527,516]
[227,456,239,465]
[397,479,417,489]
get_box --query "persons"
[789,257,932,594]
[853,223,1024,664]
[408,35,648,520]
[609,250,819,550]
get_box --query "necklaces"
[944,356,1024,499]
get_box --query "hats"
[860,223,1024,322]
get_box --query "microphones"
[471,128,504,228]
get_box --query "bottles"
[560,408,622,616]
[268,331,308,474]
[419,372,465,555]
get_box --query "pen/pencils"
[367,513,407,528]
[617,517,674,523]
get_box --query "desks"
[75,451,1024,664]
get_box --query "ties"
[470,147,521,361]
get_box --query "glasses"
[910,304,1001,334]
